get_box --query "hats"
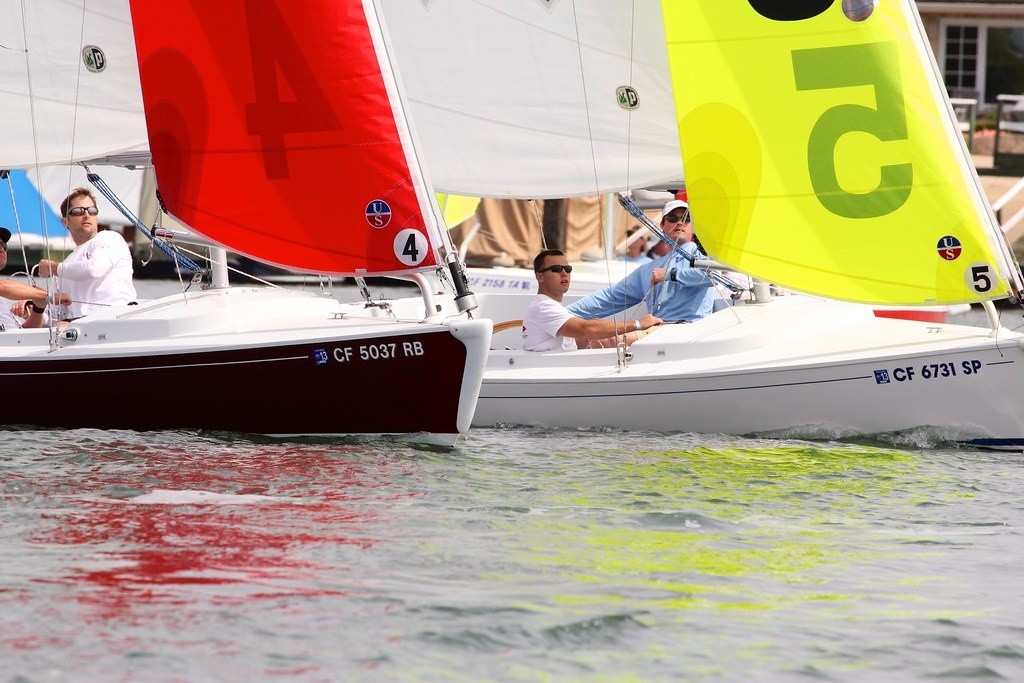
[662,200,689,221]
[0,227,11,244]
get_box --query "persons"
[0,227,71,329]
[11,188,137,329]
[617,189,706,263]
[565,200,720,348]
[522,249,663,351]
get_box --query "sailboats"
[0,0,1024,450]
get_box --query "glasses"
[540,265,572,273]
[68,206,99,215]
[0,241,8,251]
[665,215,690,223]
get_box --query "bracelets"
[45,291,52,302]
[33,305,45,313]
[635,320,641,330]
[671,268,677,282]
